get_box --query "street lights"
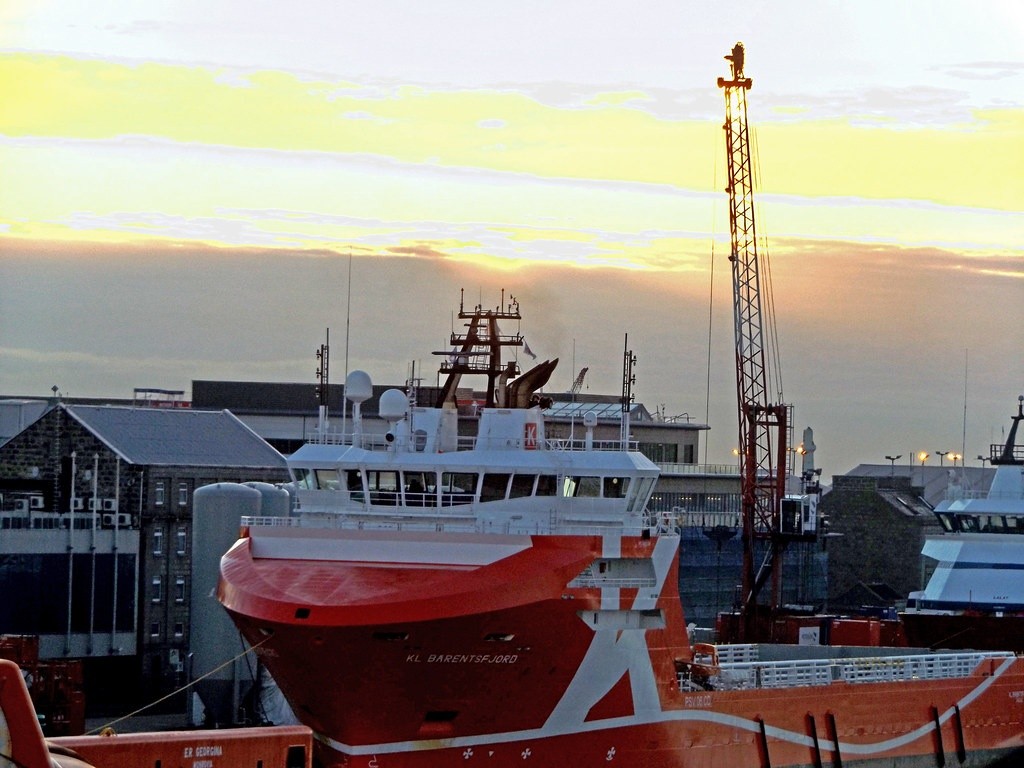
[935,451,950,466]
[731,449,744,473]
[948,453,962,466]
[976,454,991,491]
[787,445,803,475]
[884,454,903,476]
[918,451,930,486]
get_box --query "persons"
[408,479,422,506]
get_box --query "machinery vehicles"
[696,42,908,647]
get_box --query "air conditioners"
[70,498,84,509]
[104,499,116,511]
[88,499,102,510]
[30,496,45,508]
[118,514,131,525]
[14,499,29,511]
[103,514,116,525]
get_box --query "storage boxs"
[714,604,908,648]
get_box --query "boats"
[897,395,1024,657]
[0,254,1024,768]
